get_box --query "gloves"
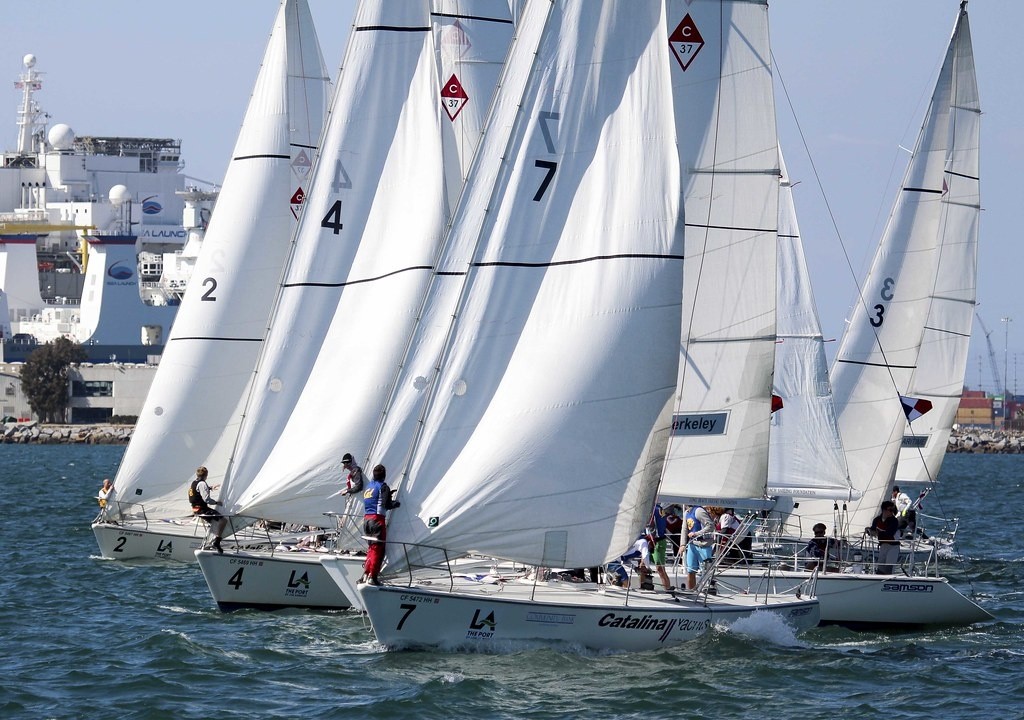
[902,510,907,517]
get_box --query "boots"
[212,537,223,554]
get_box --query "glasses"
[885,508,894,513]
[815,530,823,534]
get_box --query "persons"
[340,454,364,495]
[98,479,113,508]
[804,523,846,572]
[608,503,752,594]
[891,486,929,540]
[189,466,227,554]
[867,501,901,575]
[356,464,400,585]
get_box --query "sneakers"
[368,578,383,586]
[363,573,368,582]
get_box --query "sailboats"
[92,1,331,557]
[355,0,824,650]
[619,2,1002,625]
[193,0,524,611]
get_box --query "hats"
[341,453,352,463]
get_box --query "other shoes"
[925,540,932,544]
